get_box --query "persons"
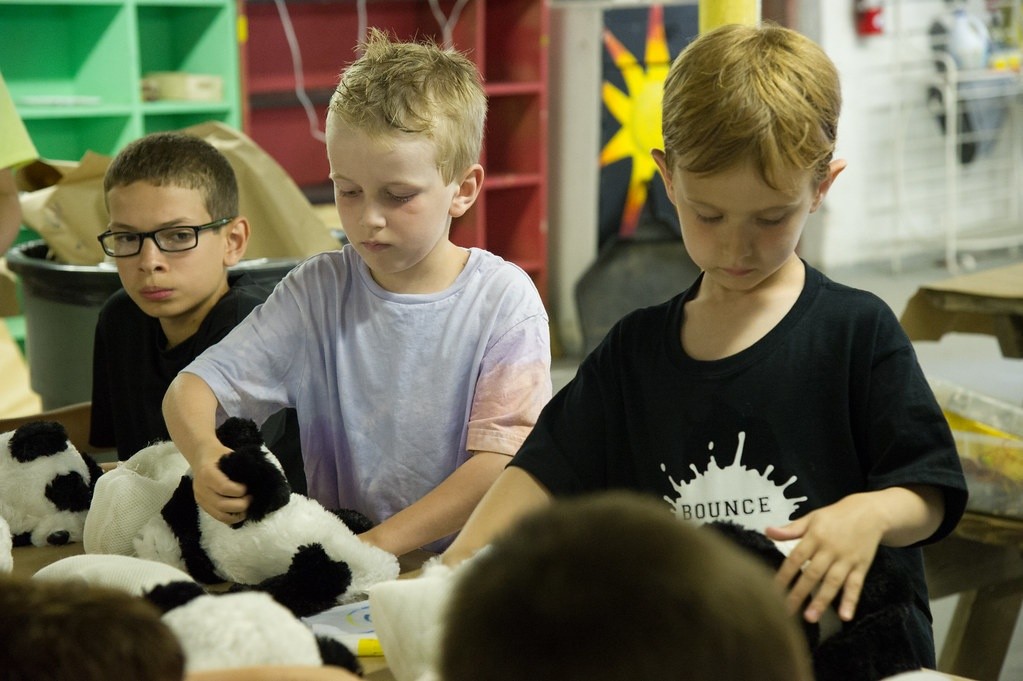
[442,25,968,681]
[437,496,813,681]
[87,134,307,495]
[161,25,552,571]
[1,71,39,257]
[0,579,360,681]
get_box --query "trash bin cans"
[3,224,354,417]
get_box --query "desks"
[899,263,1023,360]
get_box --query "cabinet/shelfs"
[0,0,242,357]
[237,0,550,315]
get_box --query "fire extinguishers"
[854,0,886,37]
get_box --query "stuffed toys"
[133,418,400,618]
[0,420,104,574]
[34,553,365,678]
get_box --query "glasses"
[97,217,233,257]
